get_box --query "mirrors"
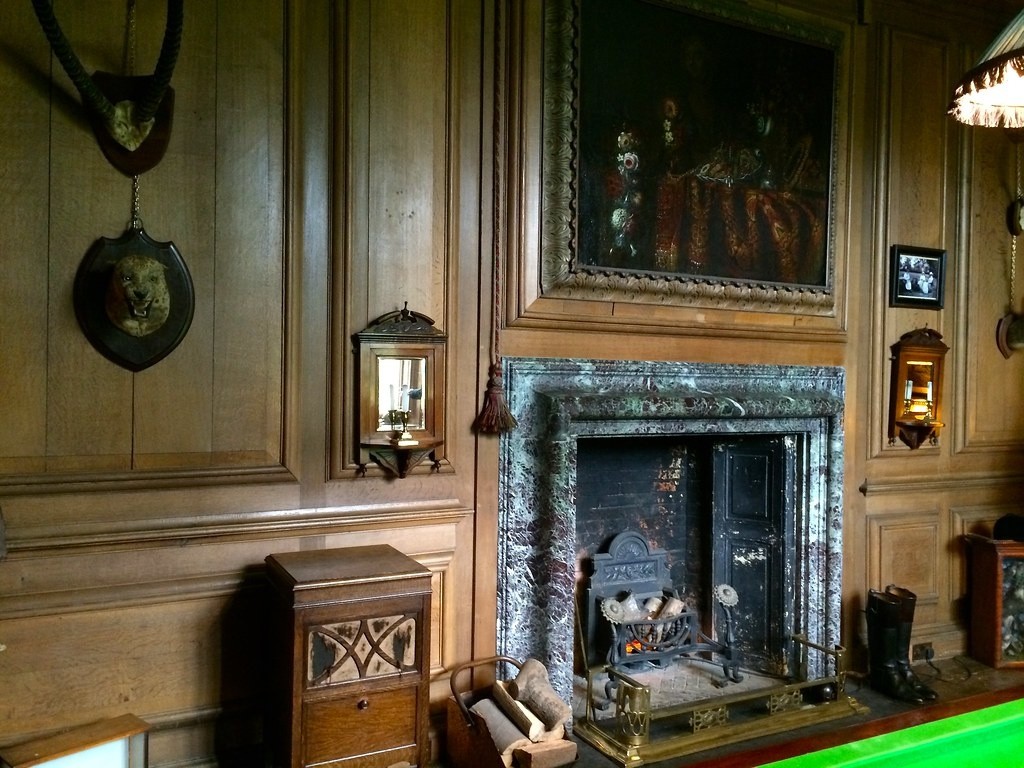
[889,326,951,450]
[352,300,448,479]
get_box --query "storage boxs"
[964,532,1024,670]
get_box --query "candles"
[906,380,913,400]
[927,381,933,401]
[401,385,410,412]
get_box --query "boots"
[884,584,939,702]
[866,589,925,706]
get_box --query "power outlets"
[912,642,932,661]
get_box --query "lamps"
[945,5,1024,129]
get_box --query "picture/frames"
[538,0,846,320]
[889,243,947,311]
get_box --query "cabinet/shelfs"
[263,543,435,768]
